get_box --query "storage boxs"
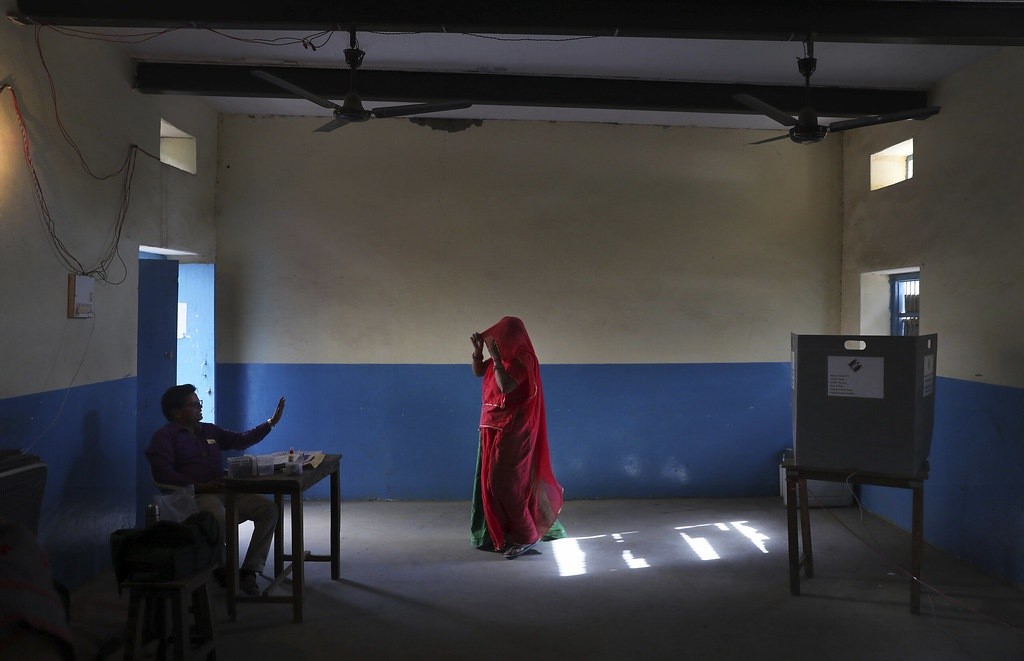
[227,456,257,480]
[256,455,275,477]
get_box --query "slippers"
[503,542,537,557]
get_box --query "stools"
[119,563,223,661]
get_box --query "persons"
[147,384,286,599]
[470,315,566,558]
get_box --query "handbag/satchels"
[110,511,224,582]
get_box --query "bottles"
[289,448,294,462]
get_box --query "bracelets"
[472,352,483,361]
[492,358,502,362]
[266,418,275,430]
[493,365,505,372]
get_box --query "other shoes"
[213,568,225,587]
[240,569,260,595]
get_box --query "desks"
[219,454,343,624]
[780,457,930,616]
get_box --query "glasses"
[182,400,203,408]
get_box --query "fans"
[732,38,941,146]
[248,25,473,133]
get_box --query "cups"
[256,455,274,476]
